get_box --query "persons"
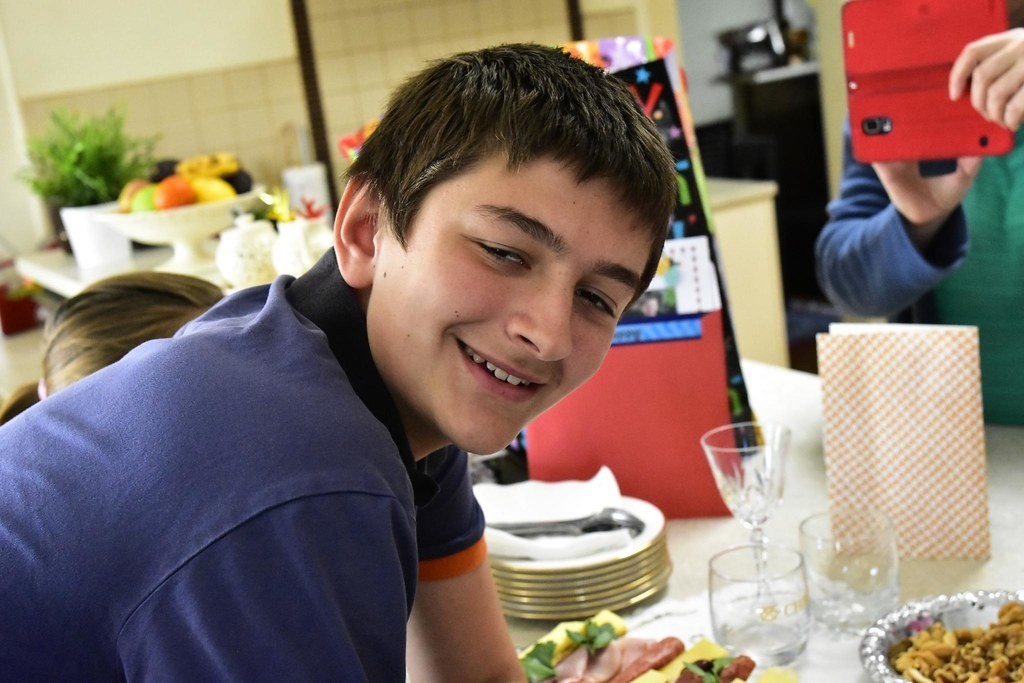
[816,0,1023,427]
[1,40,678,683]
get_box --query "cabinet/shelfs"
[703,178,792,368]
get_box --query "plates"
[486,496,672,622]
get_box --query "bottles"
[270,215,335,278]
[215,212,278,291]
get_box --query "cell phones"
[846,72,1013,164]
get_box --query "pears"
[193,176,236,203]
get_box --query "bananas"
[171,152,239,177]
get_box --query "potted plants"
[16,100,165,272]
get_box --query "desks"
[19,237,220,300]
[506,363,1024,683]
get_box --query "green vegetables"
[684,654,735,683]
[519,622,619,683]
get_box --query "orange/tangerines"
[153,174,197,209]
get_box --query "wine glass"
[700,420,794,598]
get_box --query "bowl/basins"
[858,588,1024,682]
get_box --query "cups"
[798,507,899,636]
[280,161,331,214]
[708,543,813,673]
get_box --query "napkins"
[474,464,632,559]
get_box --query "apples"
[117,176,157,211]
